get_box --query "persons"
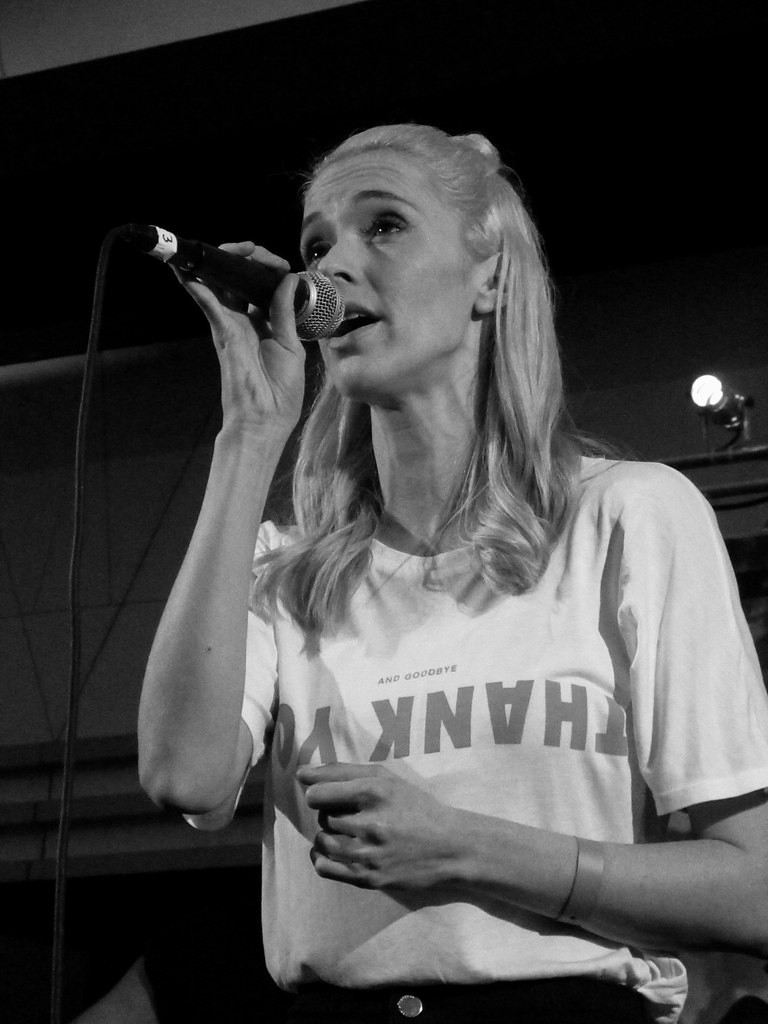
[137,124,768,1024]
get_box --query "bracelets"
[557,836,604,925]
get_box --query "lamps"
[691,372,754,451]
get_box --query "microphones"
[131,223,345,341]
